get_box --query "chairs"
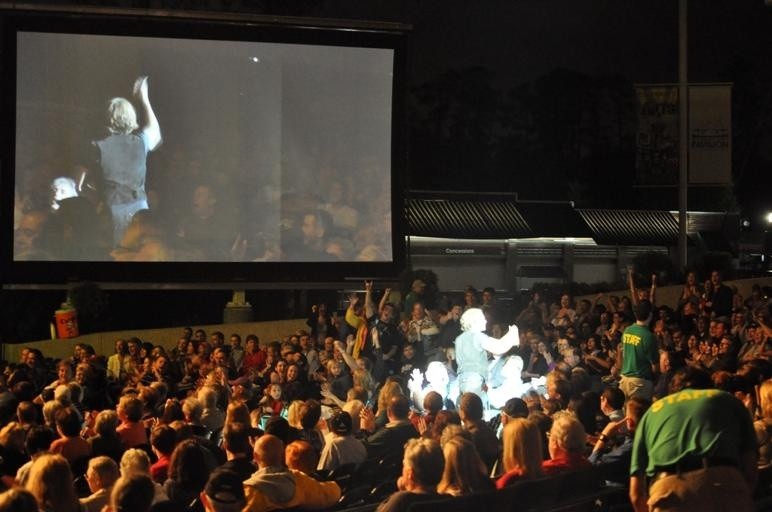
[309,449,772,512]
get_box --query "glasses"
[600,396,605,400]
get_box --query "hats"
[496,398,529,417]
[228,377,252,389]
[412,279,426,287]
[329,411,352,436]
[206,468,246,504]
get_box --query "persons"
[1,265,771,512]
[15,76,390,261]
